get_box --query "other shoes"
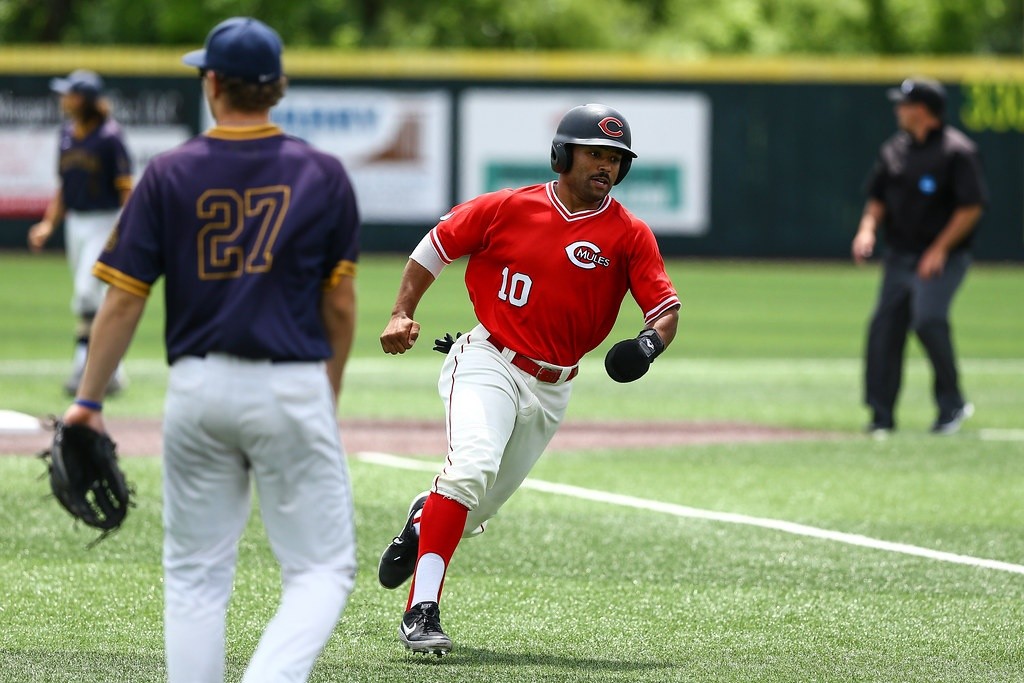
[869,411,894,433]
[934,400,974,433]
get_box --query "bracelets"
[75,397,102,412]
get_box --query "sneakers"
[398,601,452,659]
[377,491,431,589]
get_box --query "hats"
[50,70,104,99]
[886,76,947,114]
[182,16,282,80]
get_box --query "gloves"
[432,332,463,354]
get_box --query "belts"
[487,335,580,383]
[183,348,317,365]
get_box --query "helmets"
[551,104,638,186]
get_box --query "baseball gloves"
[50,423,129,529]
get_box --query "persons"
[61,17,363,683]
[381,103,682,654]
[852,76,984,438]
[28,68,134,392]
